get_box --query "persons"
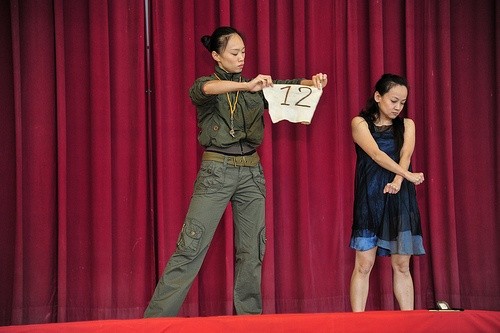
[143,27,328,317]
[350,75,424,313]
